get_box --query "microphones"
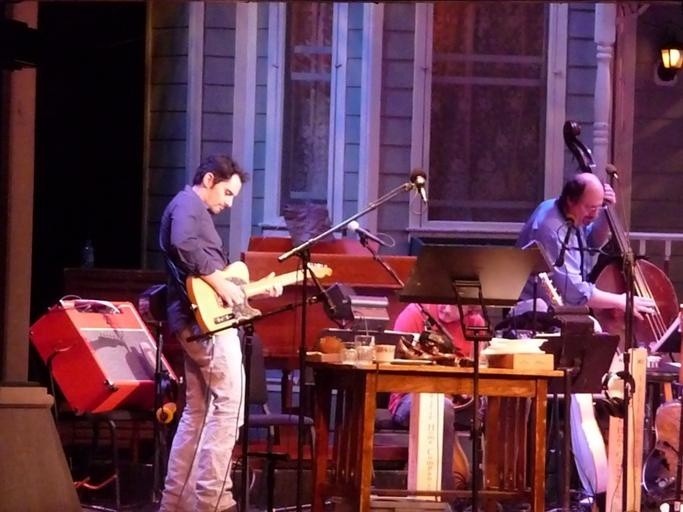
[605,164,620,182]
[555,226,571,268]
[347,220,390,247]
[410,170,426,202]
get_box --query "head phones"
[601,371,635,406]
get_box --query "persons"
[388,300,487,490]
[157,154,284,512]
[509,172,657,334]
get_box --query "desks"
[305,355,565,511]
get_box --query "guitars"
[186,261,333,333]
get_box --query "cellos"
[563,119,683,465]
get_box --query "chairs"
[242,332,316,511]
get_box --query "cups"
[338,345,357,365]
[354,334,374,366]
[374,344,395,366]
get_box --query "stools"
[646,368,680,402]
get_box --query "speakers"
[30,300,178,416]
[297,330,413,389]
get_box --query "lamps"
[655,31,683,80]
